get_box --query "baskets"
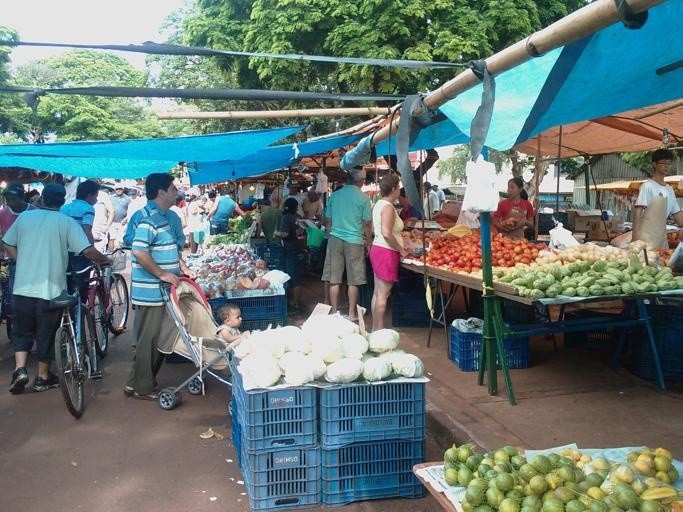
[111,252,126,271]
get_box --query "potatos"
[457,239,666,276]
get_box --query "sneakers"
[123,385,160,401]
[32,371,60,391]
[9,367,29,393]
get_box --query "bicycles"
[48,242,139,419]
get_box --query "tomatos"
[418,233,558,272]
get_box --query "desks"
[301,220,683,406]
[413,445,683,512]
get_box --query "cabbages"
[236,312,424,387]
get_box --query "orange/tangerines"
[443,444,683,512]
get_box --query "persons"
[630,147,683,251]
[493,177,536,241]
[1,168,446,400]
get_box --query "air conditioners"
[565,196,573,203]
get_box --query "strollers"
[152,274,246,411]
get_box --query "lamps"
[249,182,254,191]
[663,128,669,144]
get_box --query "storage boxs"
[588,217,624,242]
[566,208,614,233]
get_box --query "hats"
[0,182,24,196]
[113,183,125,190]
[42,183,66,200]
